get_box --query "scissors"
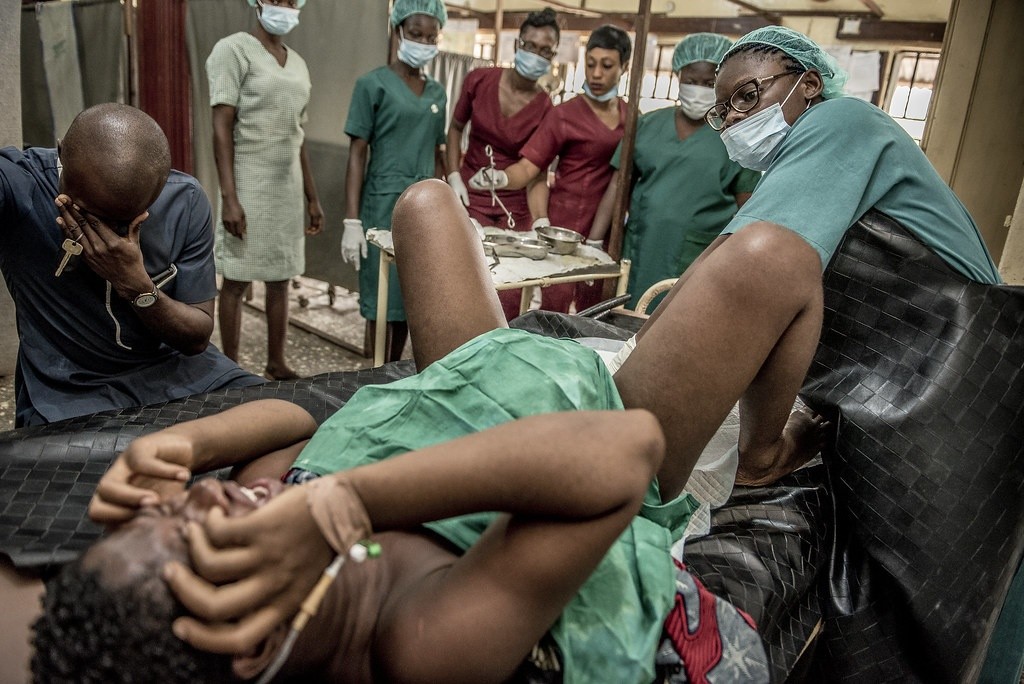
[485,145,516,228]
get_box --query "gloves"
[532,216,550,233]
[448,172,469,216]
[470,168,510,191]
[341,218,368,272]
[582,239,606,287]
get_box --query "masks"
[582,78,620,102]
[255,0,301,36]
[397,26,438,67]
[515,45,550,81]
[720,72,812,171]
[679,82,717,121]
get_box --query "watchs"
[125,284,160,307]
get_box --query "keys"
[56,239,83,277]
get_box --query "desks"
[367,229,624,365]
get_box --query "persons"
[584,33,761,314]
[469,24,642,314]
[610,26,1002,373]
[29,178,824,684]
[340,0,448,363]
[0,103,272,428]
[206,0,324,380]
[445,7,560,237]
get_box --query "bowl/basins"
[535,226,585,255]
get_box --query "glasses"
[703,70,805,132]
[521,37,558,60]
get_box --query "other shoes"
[266,364,299,383]
